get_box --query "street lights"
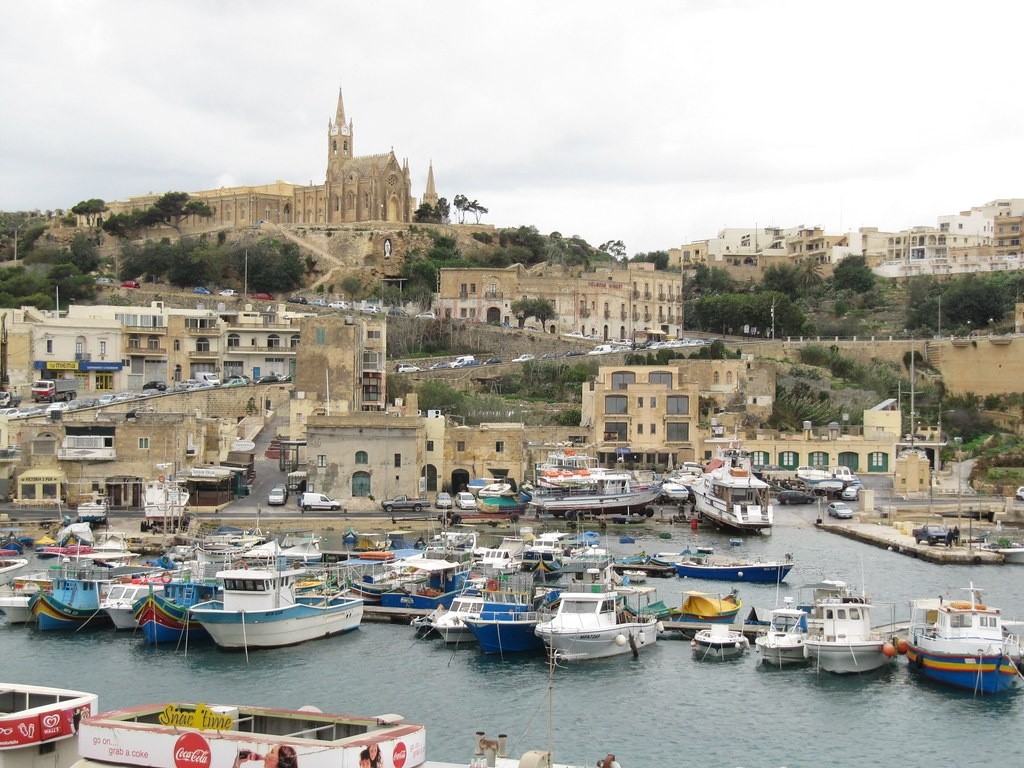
[953,437,962,545]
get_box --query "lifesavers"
[601,524,606,529]
[487,579,497,591]
[570,450,574,456]
[161,572,172,583]
[390,571,397,579]
[492,522,497,527]
[951,601,972,609]
[118,577,131,582]
[235,560,247,570]
[973,556,982,564]
[639,507,645,516]
[132,579,163,586]
[511,513,519,523]
[44,547,67,554]
[571,524,576,529]
[646,508,654,517]
[158,475,165,482]
[68,546,90,552]
[447,512,450,518]
[453,515,461,524]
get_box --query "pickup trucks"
[381,495,432,513]
[913,524,948,546]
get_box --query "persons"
[360,745,383,768]
[233,745,299,768]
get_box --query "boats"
[675,550,795,585]
[189,554,363,650]
[798,474,844,495]
[624,600,677,624]
[412,566,572,653]
[132,581,223,646]
[534,592,663,667]
[467,477,532,524]
[529,441,664,525]
[666,421,774,533]
[757,579,899,674]
[690,625,750,664]
[207,524,748,605]
[908,580,1024,696]
[669,589,742,625]
[0,462,197,630]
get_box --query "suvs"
[1016,486,1024,501]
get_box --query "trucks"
[30,378,85,404]
[0,393,23,409]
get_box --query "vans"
[300,492,341,512]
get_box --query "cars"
[435,492,477,510]
[828,502,853,519]
[759,464,865,501]
[680,461,704,474]
[0,275,724,421]
[268,482,288,507]
[776,489,816,504]
